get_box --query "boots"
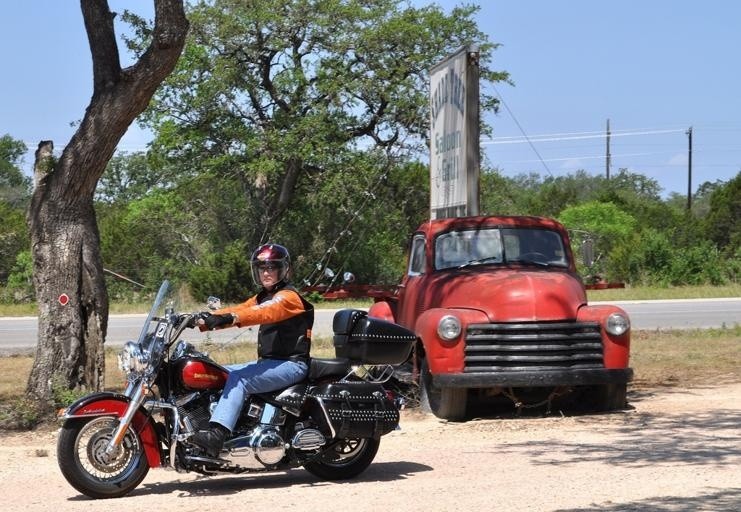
[187,423,230,459]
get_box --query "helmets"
[249,244,291,288]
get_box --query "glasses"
[258,266,281,274]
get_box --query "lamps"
[304,267,356,289]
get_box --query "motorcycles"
[56,276,417,497]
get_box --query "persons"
[174,244,315,457]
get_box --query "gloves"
[205,313,234,331]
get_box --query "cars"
[367,215,631,421]
